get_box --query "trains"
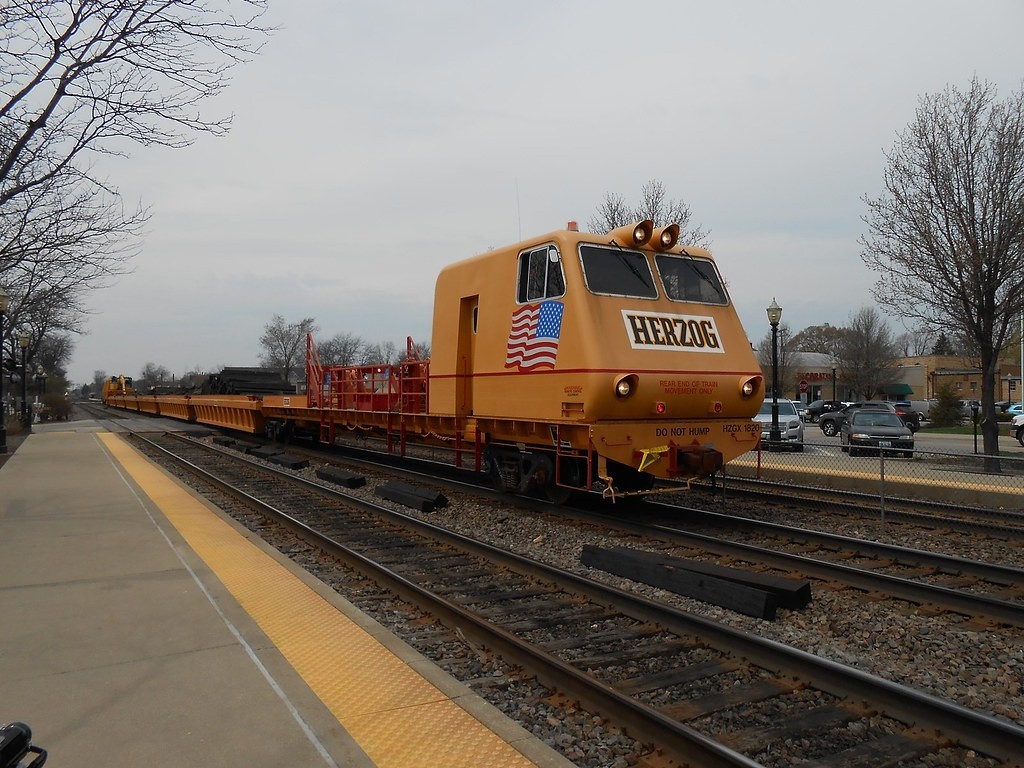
[100,219,765,501]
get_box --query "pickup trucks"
[954,399,1001,418]
[911,399,942,421]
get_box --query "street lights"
[0,287,9,454]
[1007,373,1013,405]
[17,333,29,419]
[765,296,782,442]
[36,364,44,394]
[830,358,838,402]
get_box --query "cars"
[751,397,805,451]
[804,400,914,458]
[994,400,1024,447]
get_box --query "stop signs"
[799,380,809,392]
